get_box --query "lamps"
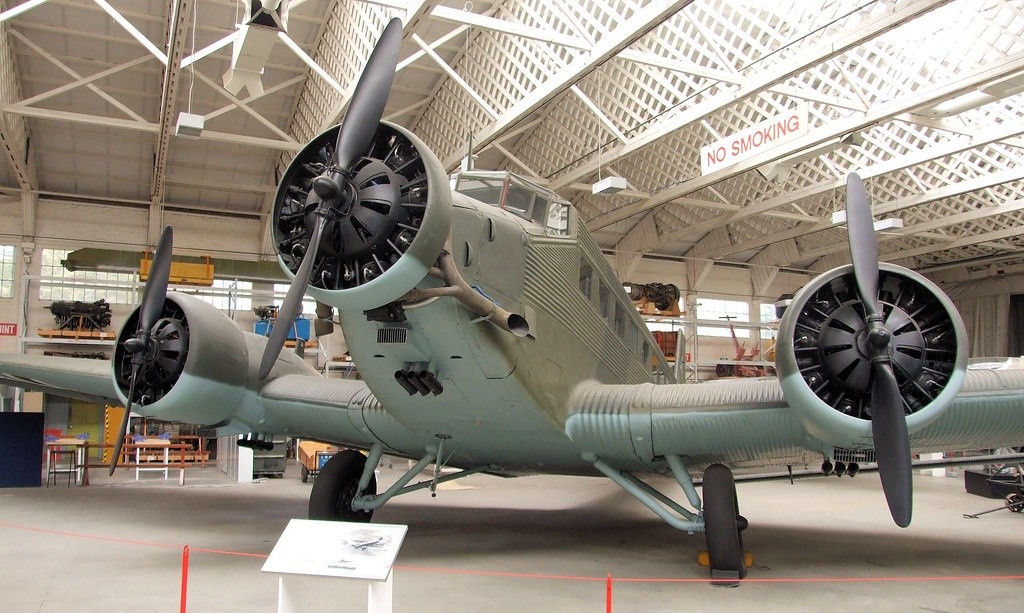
[176,112,205,138]
[593,177,627,196]
[831,210,847,225]
[874,218,904,231]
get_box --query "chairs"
[47,449,78,488]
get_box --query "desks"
[125,434,215,468]
[45,439,86,485]
[76,441,193,487]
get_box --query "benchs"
[75,464,192,468]
[121,451,211,455]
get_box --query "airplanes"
[0,12,1022,584]
